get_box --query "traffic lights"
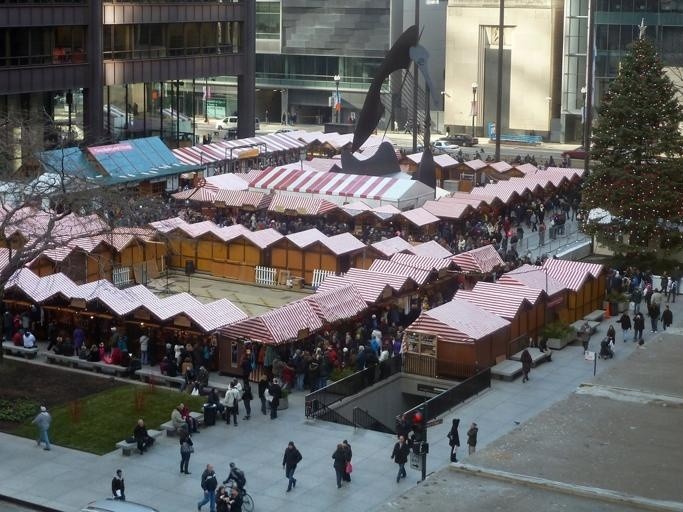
[411,408,426,442]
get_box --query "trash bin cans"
[609,303,618,316]
[204,403,217,425]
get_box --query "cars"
[416,140,460,153]
[439,134,478,147]
[600,148,655,166]
[561,147,600,160]
[78,498,159,512]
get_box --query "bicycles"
[223,480,254,512]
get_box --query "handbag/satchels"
[180,444,194,453]
[346,463,352,473]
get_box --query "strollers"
[598,336,614,360]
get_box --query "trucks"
[216,116,261,132]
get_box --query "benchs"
[490,310,608,381]
[1,340,240,456]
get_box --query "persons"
[394,119,450,136]
[282,441,302,492]
[198,462,246,512]
[32,406,50,450]
[213,149,572,176]
[179,435,193,474]
[57,186,582,254]
[134,420,153,455]
[241,246,548,419]
[331,440,352,488]
[203,132,217,144]
[391,414,410,483]
[112,469,125,500]
[448,420,478,463]
[172,378,244,436]
[14,312,218,392]
[580,266,675,359]
[267,112,297,125]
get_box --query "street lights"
[580,86,586,146]
[471,82,478,137]
[334,75,341,123]
